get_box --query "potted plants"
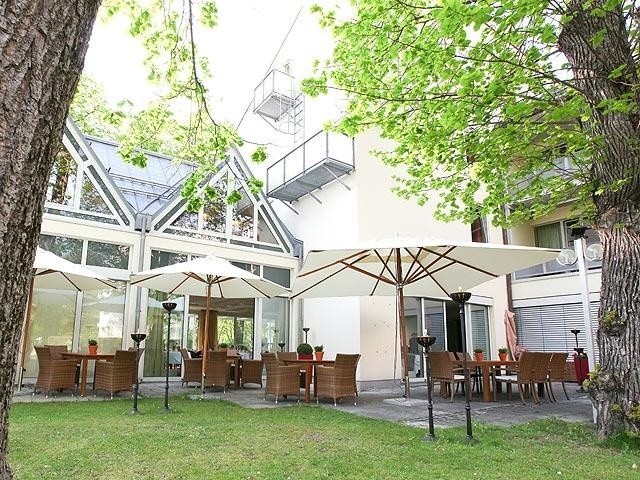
[474,349,485,361]
[497,348,508,361]
[314,345,324,361]
[297,344,314,383]
[87,339,98,354]
[220,343,227,352]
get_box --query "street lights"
[557,227,605,424]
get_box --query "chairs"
[316,353,362,405]
[107,347,145,384]
[276,351,310,388]
[445,350,460,374]
[219,349,237,363]
[32,346,78,398]
[547,352,569,401]
[179,349,203,387]
[241,351,268,389]
[260,352,300,404]
[456,352,492,392]
[531,351,554,404]
[428,351,473,404]
[44,344,80,389]
[494,351,537,406]
[93,350,137,399]
[202,350,231,393]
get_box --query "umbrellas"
[288,234,562,400]
[128,255,292,395]
[15,246,118,391]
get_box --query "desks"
[60,351,115,397]
[456,360,517,401]
[199,354,243,390]
[284,359,334,403]
